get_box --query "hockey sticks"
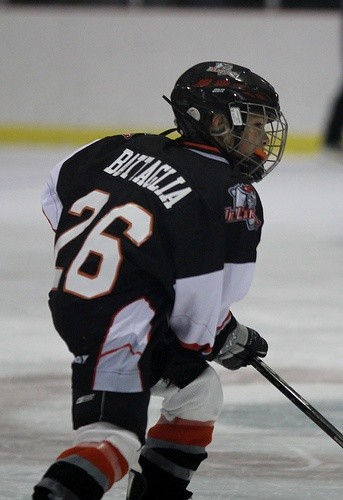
[250,354,343,449]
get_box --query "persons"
[31,60,289,500]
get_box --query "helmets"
[170,61,280,145]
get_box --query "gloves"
[209,322,268,371]
